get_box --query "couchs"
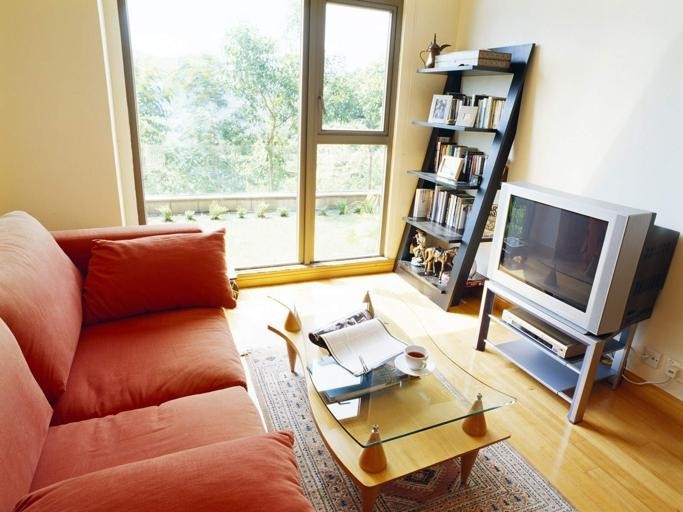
[1,209,314,512]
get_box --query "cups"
[405,345,429,371]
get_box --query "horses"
[424,246,456,281]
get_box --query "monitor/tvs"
[486,178,681,337]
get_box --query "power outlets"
[640,345,683,384]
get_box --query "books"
[308,309,408,376]
[305,355,420,402]
[411,93,506,239]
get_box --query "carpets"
[245,345,580,512]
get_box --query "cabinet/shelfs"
[473,282,637,422]
[395,42,535,312]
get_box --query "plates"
[395,351,437,377]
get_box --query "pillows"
[81,226,236,326]
[13,426,314,512]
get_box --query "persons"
[412,229,426,261]
